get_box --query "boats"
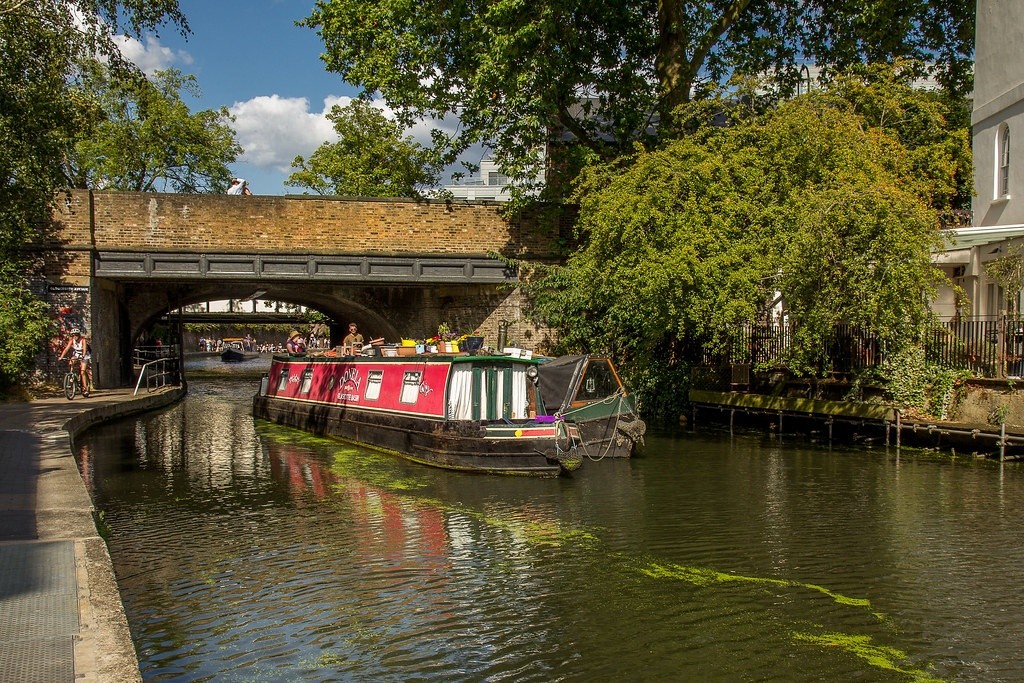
[221,346,259,360]
[537,348,645,459]
[253,322,583,478]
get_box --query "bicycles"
[56,355,91,400]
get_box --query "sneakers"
[69,378,74,383]
[84,391,90,395]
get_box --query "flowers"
[459,334,467,340]
[466,326,480,336]
[447,332,459,340]
[414,337,425,345]
[438,322,450,341]
[432,336,439,346]
[425,338,432,346]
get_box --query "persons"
[196,333,335,355]
[242,181,252,195]
[227,177,246,196]
[57,328,91,396]
[343,323,365,357]
[287,330,306,357]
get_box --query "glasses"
[72,334,79,337]
[350,328,357,332]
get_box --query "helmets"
[70,328,81,335]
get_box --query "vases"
[444,342,452,353]
[416,345,425,353]
[460,340,467,351]
[440,341,446,353]
[426,346,430,351]
[467,337,483,350]
[430,345,438,353]
[450,341,460,352]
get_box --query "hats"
[289,330,302,340]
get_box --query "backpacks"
[86,343,92,354]
